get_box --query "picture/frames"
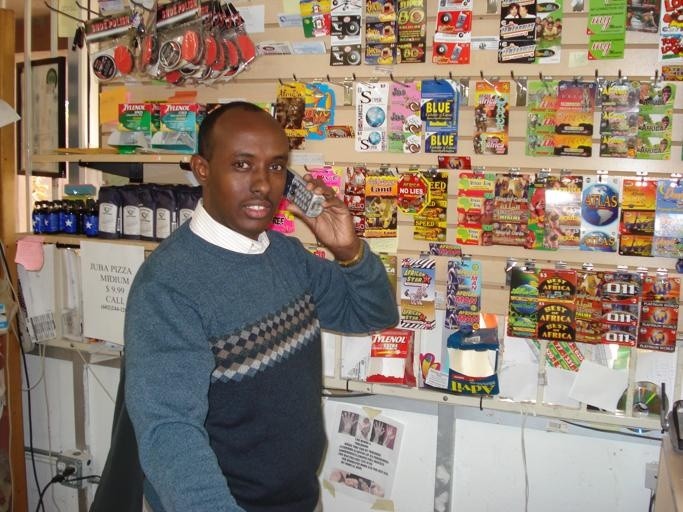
[14,56,66,178]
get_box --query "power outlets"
[55,452,92,491]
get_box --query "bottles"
[32,198,100,238]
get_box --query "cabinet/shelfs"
[16,151,200,252]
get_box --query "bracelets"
[336,239,364,266]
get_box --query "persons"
[121,100,400,512]
[517,5,535,18]
[534,16,562,41]
[329,470,385,498]
[340,411,396,447]
[502,3,520,19]
[29,67,58,176]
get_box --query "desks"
[652,437,683,512]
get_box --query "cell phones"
[282,168,326,218]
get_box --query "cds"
[636,381,669,417]
[616,389,662,433]
